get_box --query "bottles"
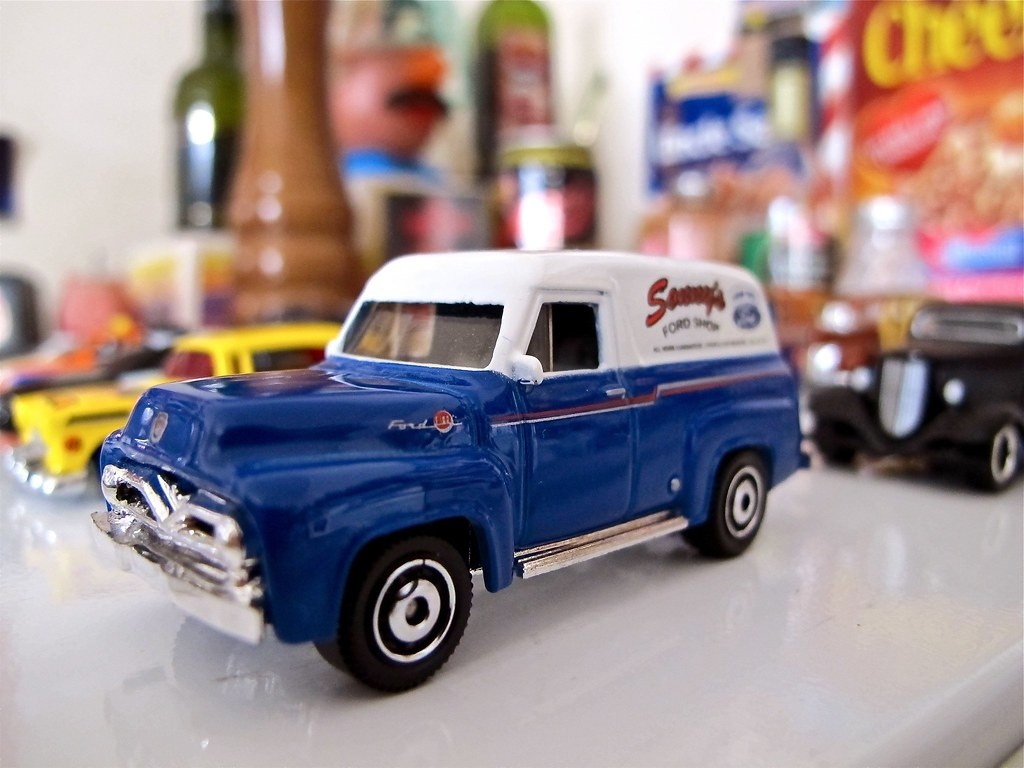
[845,198,930,362]
[499,147,598,251]
[175,0,248,231]
[0,135,43,440]
[641,36,842,371]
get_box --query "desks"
[0,444,1024,768]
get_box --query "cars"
[79,244,816,694]
[805,298,1024,492]
[0,305,391,502]
